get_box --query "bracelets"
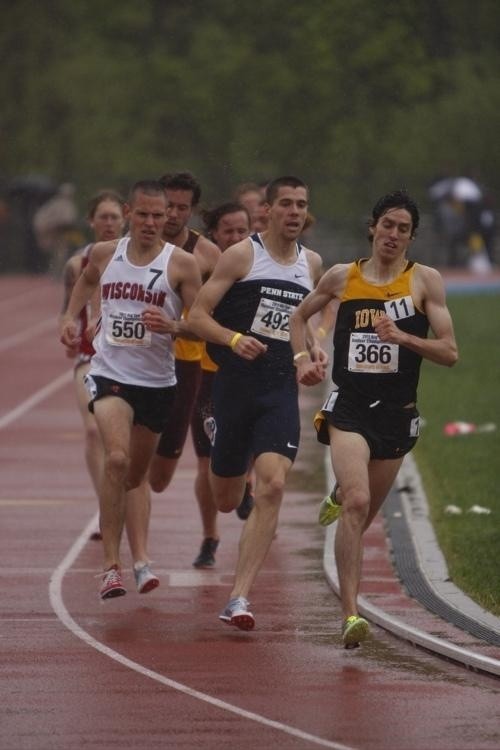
[314,324,330,344]
[293,350,309,365]
[227,330,244,350]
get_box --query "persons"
[62,178,204,601]
[289,190,459,652]
[193,186,324,632]
[0,163,499,289]
[191,199,253,572]
[146,172,222,568]
[59,193,128,539]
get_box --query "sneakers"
[318,482,343,526]
[218,594,256,631]
[94,564,127,599]
[342,615,369,649]
[236,477,256,520]
[91,527,102,540]
[194,532,220,569]
[133,563,160,594]
